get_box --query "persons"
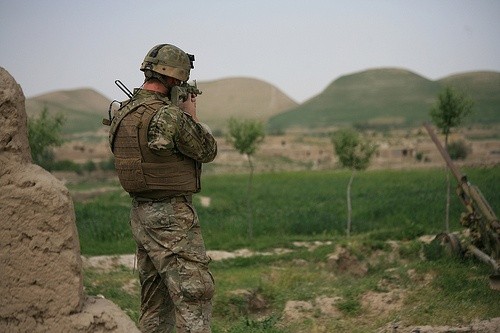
[102,43,220,333]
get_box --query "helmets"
[139,43,191,81]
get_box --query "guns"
[171,78,203,108]
